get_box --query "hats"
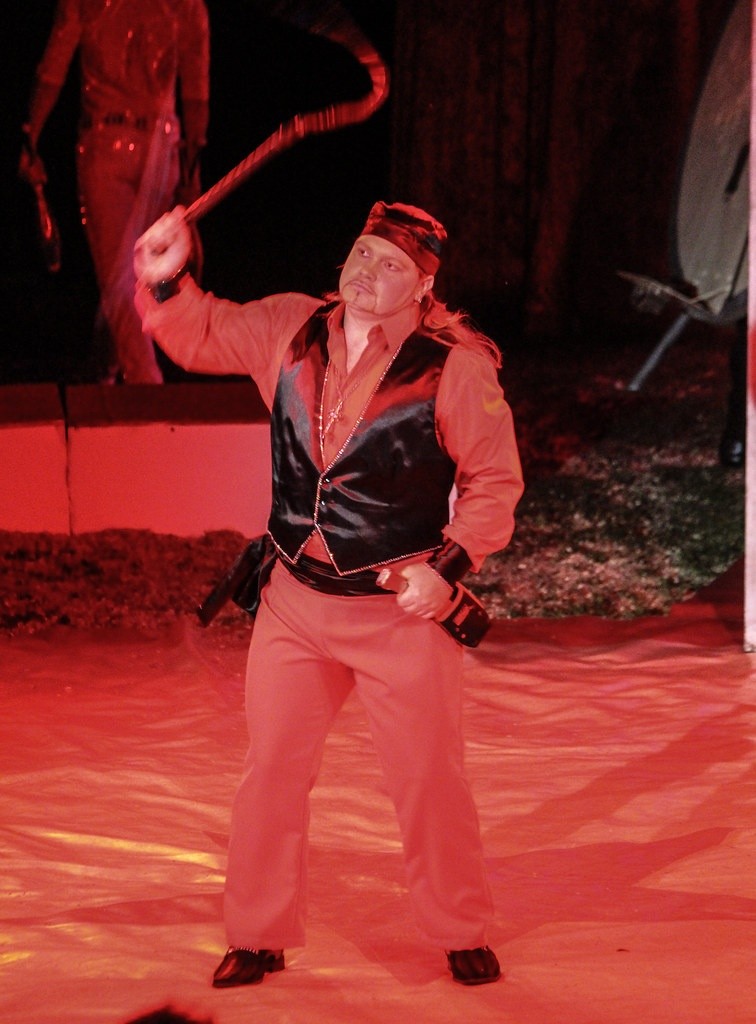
[359,199,449,281]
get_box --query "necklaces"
[321,360,361,435]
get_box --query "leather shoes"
[210,946,284,989]
[444,944,500,985]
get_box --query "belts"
[86,113,147,129]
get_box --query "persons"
[17,0,210,386]
[132,204,524,989]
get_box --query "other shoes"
[102,368,163,384]
